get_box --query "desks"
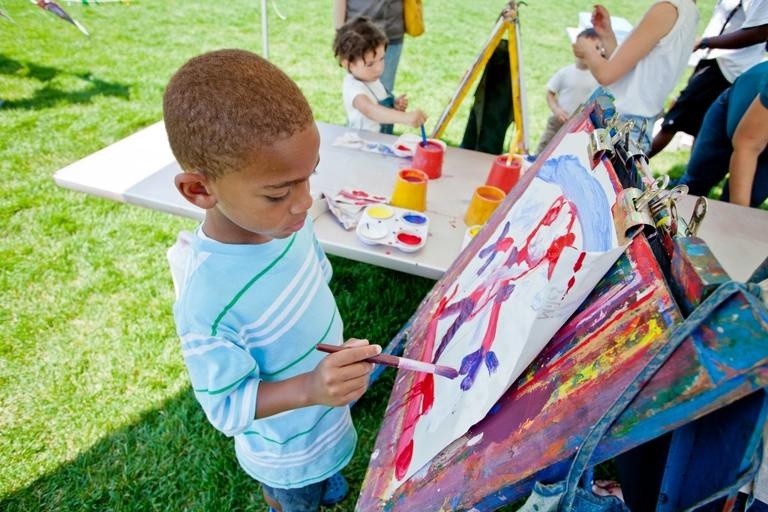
[52,116,768,287]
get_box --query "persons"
[330,15,429,137]
[160,47,385,511]
[677,59,768,208]
[647,0,768,161]
[333,0,405,135]
[571,0,703,182]
[532,27,603,158]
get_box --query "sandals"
[321,471,350,505]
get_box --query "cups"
[485,156,521,195]
[391,169,427,212]
[413,141,445,180]
[464,186,506,230]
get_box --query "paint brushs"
[506,131,522,167]
[421,125,428,149]
[316,344,457,379]
